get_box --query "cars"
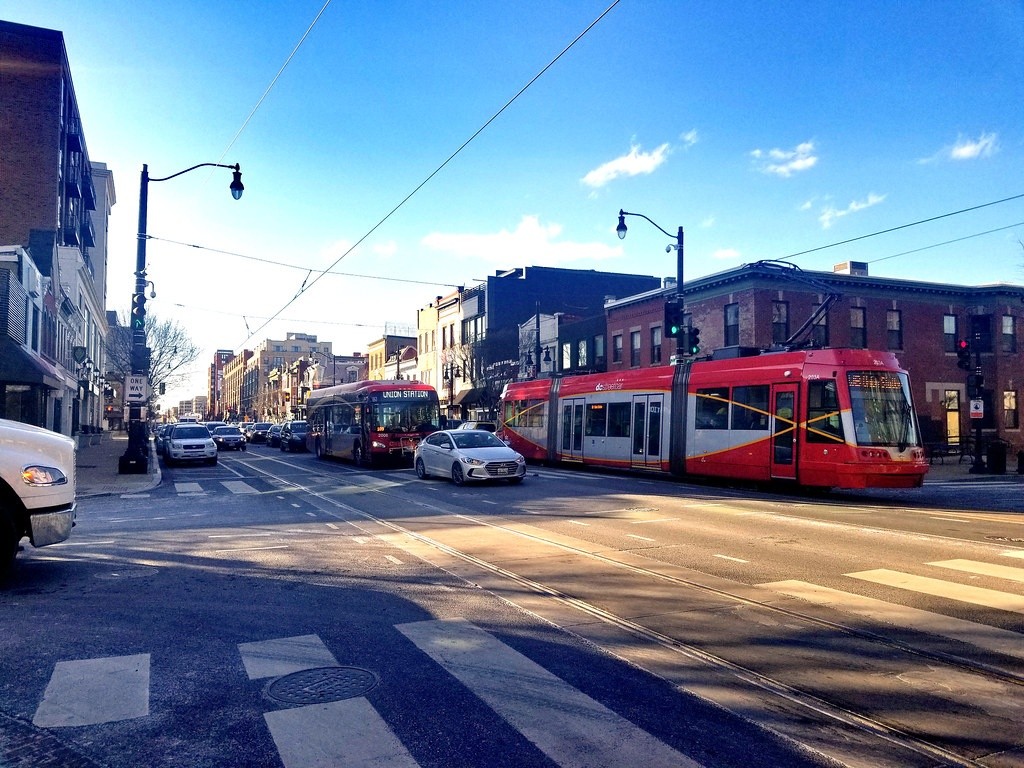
[154,416,218,467]
[414,430,528,485]
[224,422,259,440]
[267,424,287,449]
[209,426,247,451]
[249,423,277,443]
[278,421,312,452]
[206,422,226,437]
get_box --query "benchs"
[925,442,974,465]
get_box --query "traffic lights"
[688,328,701,355]
[676,329,685,355]
[661,301,682,339]
[957,338,971,370]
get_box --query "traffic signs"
[125,376,147,403]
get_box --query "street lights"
[307,350,341,386]
[131,162,244,455]
[524,298,553,380]
[615,208,686,328]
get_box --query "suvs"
[0,417,81,571]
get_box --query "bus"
[494,346,930,494]
[304,379,449,471]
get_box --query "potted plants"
[80,425,104,447]
